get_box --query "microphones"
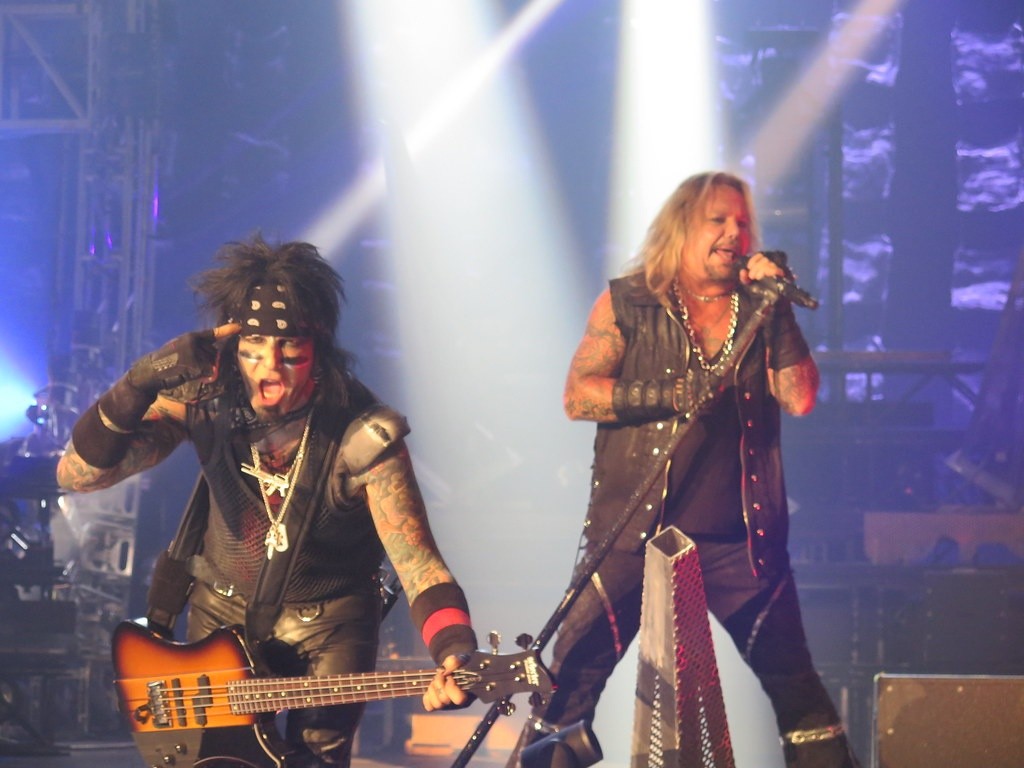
[731,255,818,311]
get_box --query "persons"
[56,242,478,768]
[509,169,853,768]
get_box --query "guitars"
[110,617,562,768]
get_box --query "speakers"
[870,671,1024,768]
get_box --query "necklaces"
[249,390,328,557]
[240,420,299,497]
[674,272,739,372]
[678,283,735,304]
